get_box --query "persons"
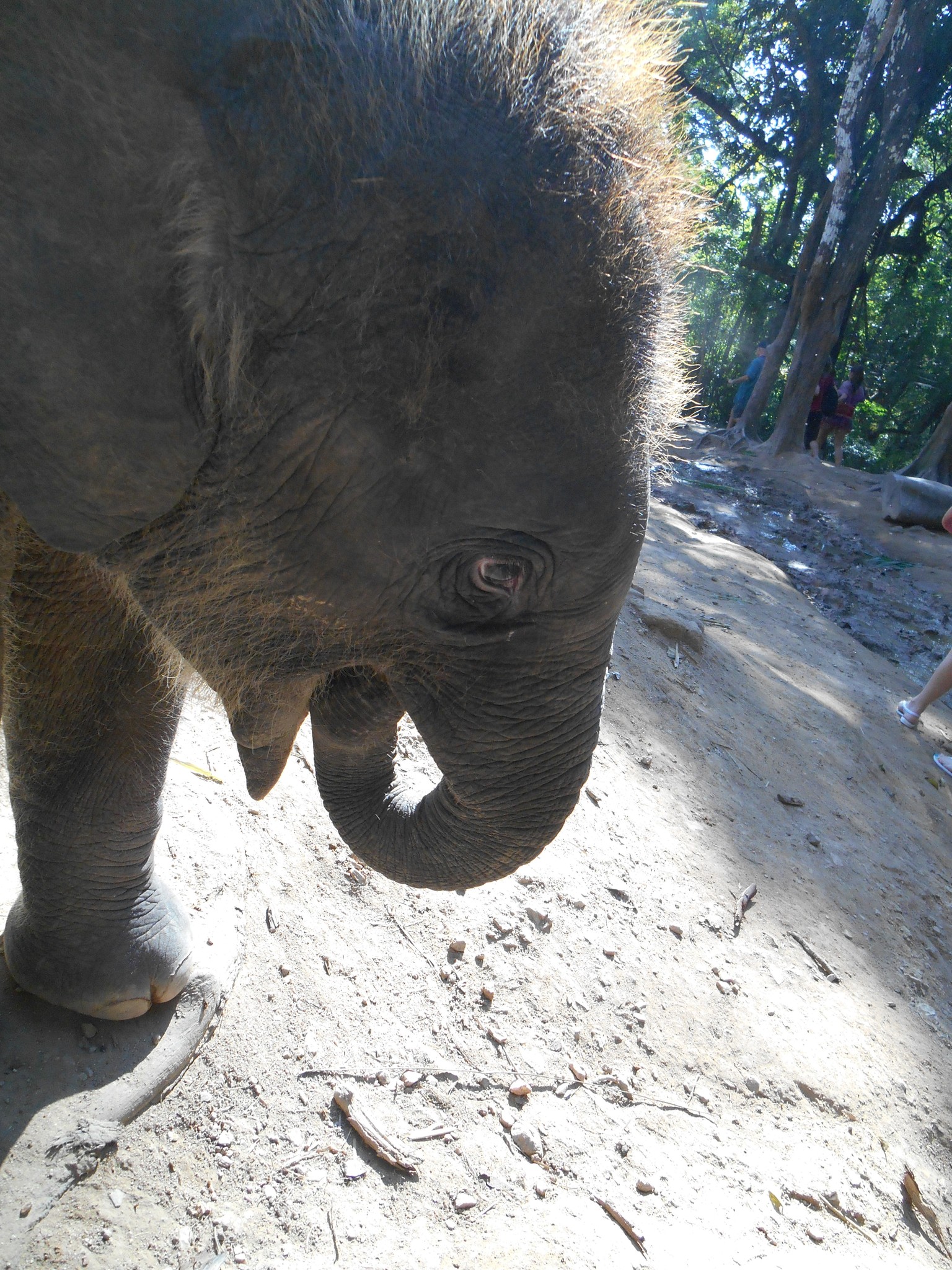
[726,337,780,440]
[803,354,844,466]
[807,361,876,467]
[897,491,952,783]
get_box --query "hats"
[754,342,768,349]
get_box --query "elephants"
[0,0,726,1024]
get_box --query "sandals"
[934,754,952,777]
[897,701,921,728]
